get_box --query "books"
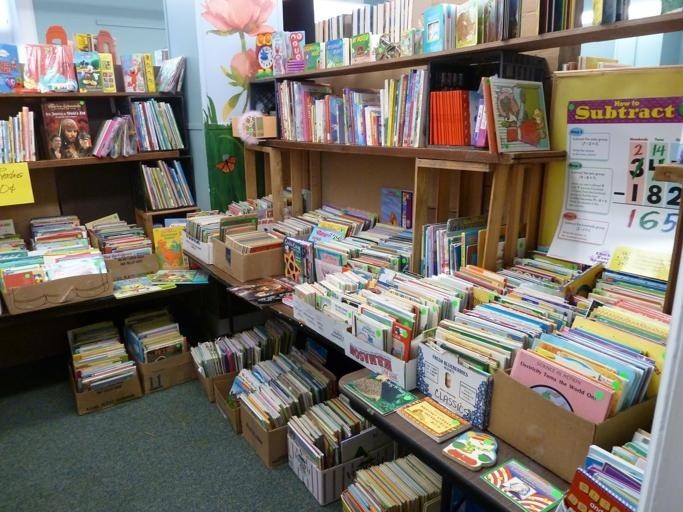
[277,69,550,153]
[185,187,674,511]
[0,213,208,300]
[0,33,187,164]
[141,160,195,210]
[254,1,629,84]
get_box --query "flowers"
[198,0,275,124]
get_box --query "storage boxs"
[171,222,296,285]
[63,337,195,417]
[291,291,657,485]
[0,233,161,316]
[182,341,392,505]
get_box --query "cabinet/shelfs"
[0,64,203,401]
[183,12,681,512]
